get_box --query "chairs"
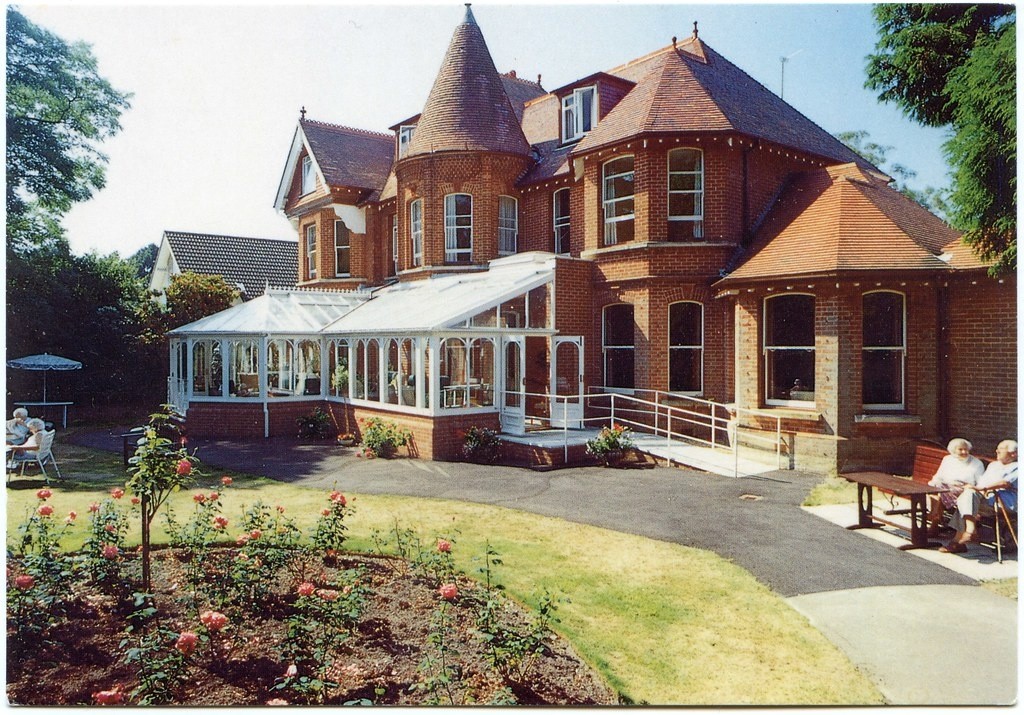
[6,422,62,485]
[121,415,187,466]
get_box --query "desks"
[6,444,18,483]
[837,471,952,552]
[13,402,74,429]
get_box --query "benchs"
[878,445,1018,552]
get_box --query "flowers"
[589,424,634,456]
[337,434,355,441]
[461,425,503,460]
[295,407,328,428]
[353,417,412,459]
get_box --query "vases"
[466,448,499,465]
[373,441,395,458]
[339,439,352,446]
[308,424,318,433]
[603,450,626,466]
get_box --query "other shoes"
[940,541,967,553]
[927,512,944,522]
[927,529,939,538]
[958,532,979,544]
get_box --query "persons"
[923,438,984,538]
[6,408,31,446]
[938,440,1017,553]
[11,417,47,460]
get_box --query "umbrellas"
[6,353,82,421]
